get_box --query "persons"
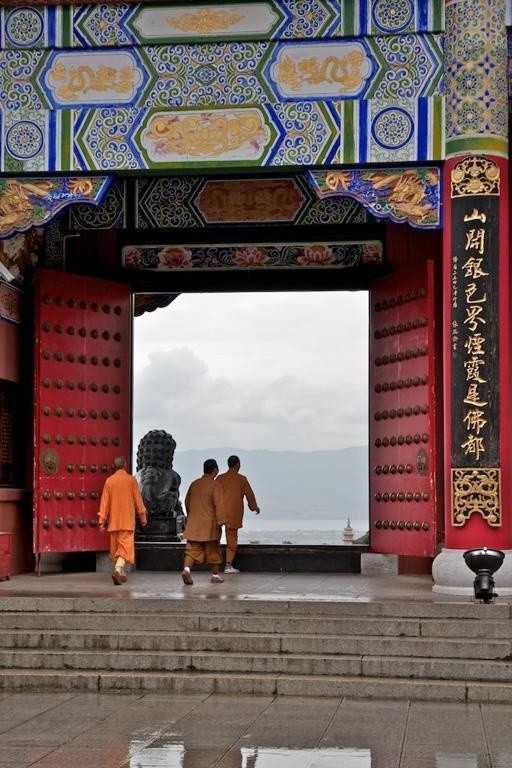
[181,459,227,585]
[97,456,147,584]
[215,455,260,573]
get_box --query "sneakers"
[224,567,240,573]
[211,576,224,583]
[181,570,193,585]
[112,571,127,585]
